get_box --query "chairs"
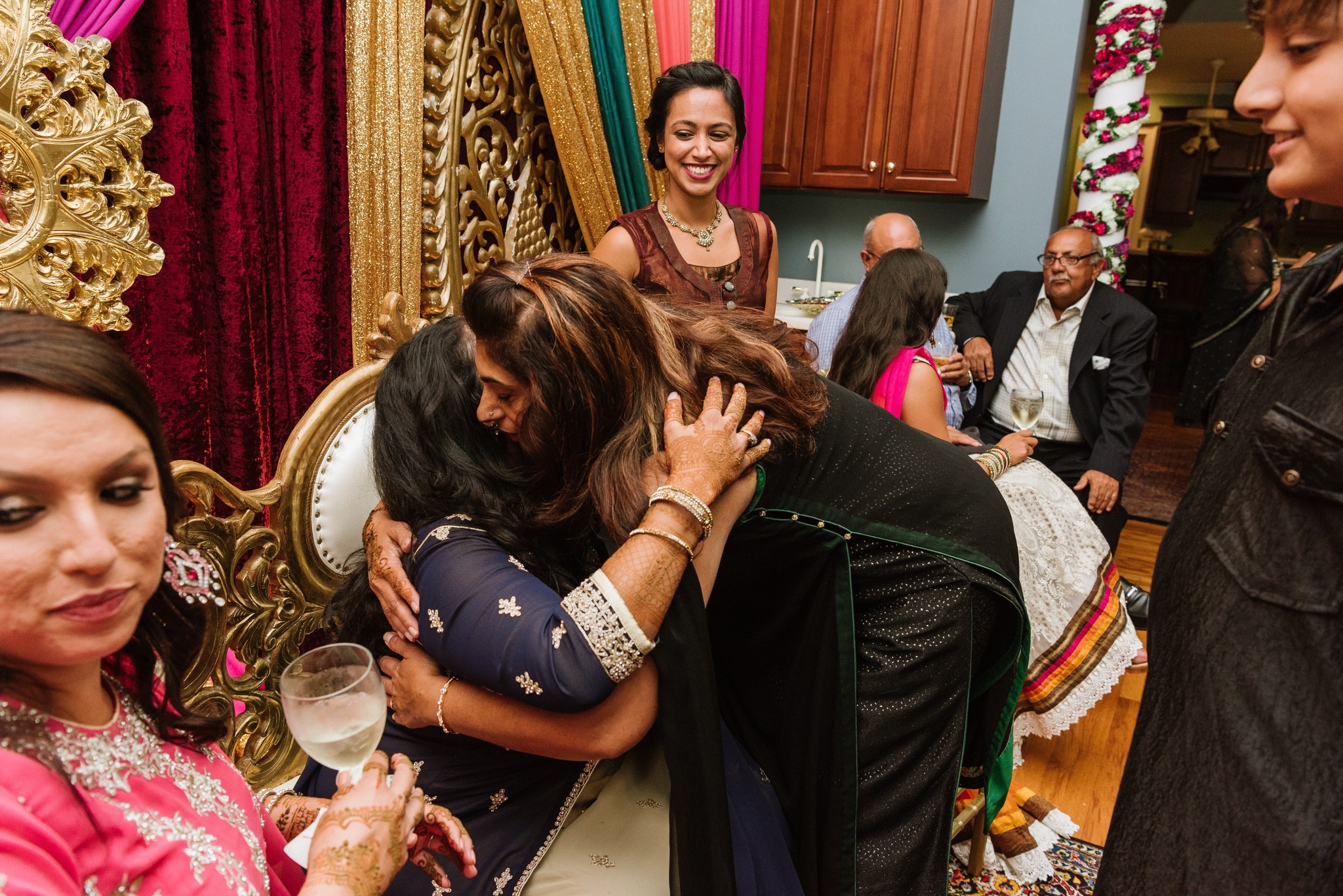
[159,287,441,816]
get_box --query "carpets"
[945,826,1105,896]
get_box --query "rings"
[737,429,757,448]
[388,698,395,709]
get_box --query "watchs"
[959,369,972,393]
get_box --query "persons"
[589,58,783,334]
[826,243,1158,778]
[801,212,1158,630]
[951,224,1158,565]
[1080,0,1342,896]
[360,250,1034,895]
[1168,169,1316,441]
[1,307,482,895]
[292,304,808,896]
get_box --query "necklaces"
[662,190,723,253]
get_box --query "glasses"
[868,245,926,261]
[1037,252,1102,266]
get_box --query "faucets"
[807,239,824,298]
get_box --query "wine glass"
[1009,389,1044,431]
[278,643,388,786]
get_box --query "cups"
[928,341,953,368]
[943,304,957,324]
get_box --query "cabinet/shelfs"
[754,0,1015,205]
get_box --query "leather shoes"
[1116,575,1151,630]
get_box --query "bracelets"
[648,485,714,542]
[628,528,694,564]
[262,788,305,818]
[426,835,436,849]
[436,675,466,735]
[973,445,1013,481]
[255,787,277,811]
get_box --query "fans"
[1138,56,1264,155]
